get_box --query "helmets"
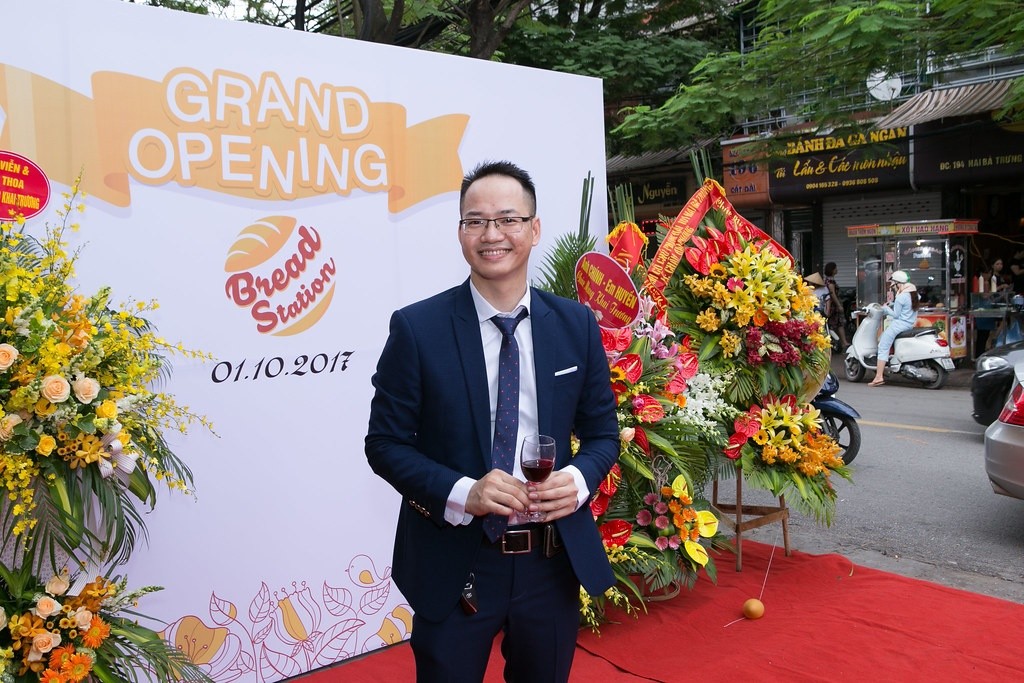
[889,271,908,284]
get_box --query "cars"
[970,340,1024,500]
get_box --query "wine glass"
[516,434,557,523]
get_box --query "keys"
[460,573,478,615]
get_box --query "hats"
[804,272,825,286]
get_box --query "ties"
[482,307,529,544]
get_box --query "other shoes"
[842,343,852,350]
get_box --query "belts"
[482,527,546,557]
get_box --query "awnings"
[867,72,1024,132]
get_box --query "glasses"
[457,215,535,235]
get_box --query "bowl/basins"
[1010,298,1024,305]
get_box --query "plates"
[951,324,966,347]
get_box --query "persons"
[824,262,851,348]
[803,272,831,318]
[985,257,1012,351]
[868,270,921,386]
[365,159,621,683]
[974,265,992,359]
[1009,246,1024,295]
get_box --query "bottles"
[991,274,997,292]
[978,274,985,293]
[972,274,978,292]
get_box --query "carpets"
[285,537,1024,683]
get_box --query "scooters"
[845,302,956,390]
[805,304,862,469]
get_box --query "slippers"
[865,381,886,387]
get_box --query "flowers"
[0,170,225,683]
[528,148,853,636]
